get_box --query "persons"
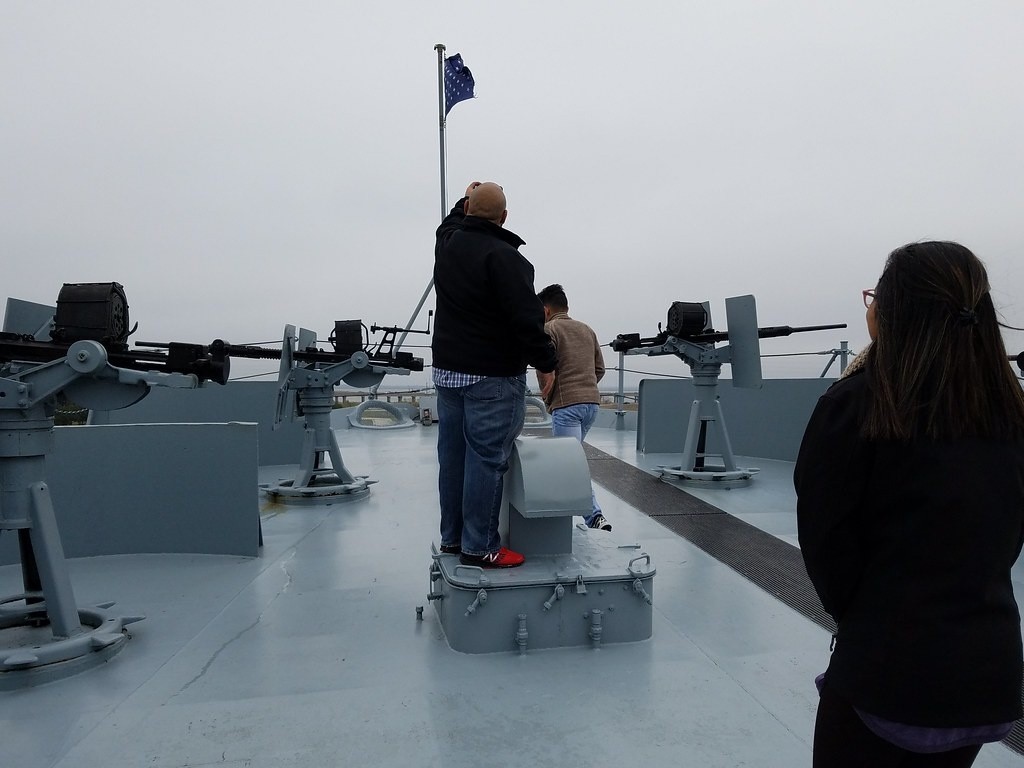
[537,284,612,531]
[792,241,1024,768]
[433,182,556,568]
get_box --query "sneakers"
[591,514,612,532]
[460,544,525,569]
[440,541,461,553]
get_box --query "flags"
[444,54,475,117]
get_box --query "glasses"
[863,288,877,309]
